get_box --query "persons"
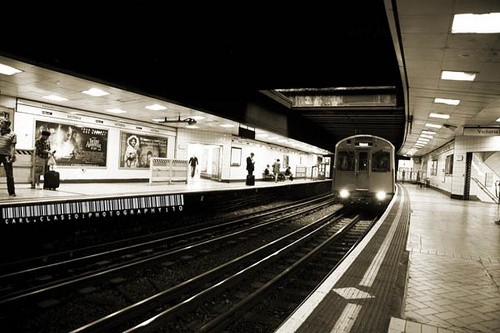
[246,153,255,175]
[278,170,285,181]
[495,184,500,225]
[34,130,55,190]
[417,171,420,186]
[124,135,139,167]
[0,116,17,197]
[286,166,293,181]
[145,151,152,166]
[265,164,275,179]
[271,159,281,183]
[402,170,405,184]
[189,154,199,177]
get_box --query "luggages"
[246,175,255,185]
[45,155,59,190]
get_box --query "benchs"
[262,172,289,181]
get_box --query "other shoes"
[37,185,42,190]
[9,194,16,197]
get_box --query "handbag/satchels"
[47,156,56,165]
[5,134,16,162]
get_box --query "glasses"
[0,122,3,124]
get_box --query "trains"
[332,134,397,209]
